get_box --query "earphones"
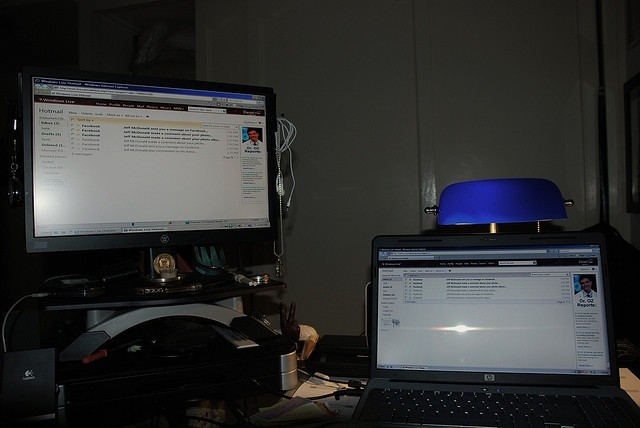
[276,178,286,196]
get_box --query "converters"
[41,275,108,298]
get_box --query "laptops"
[348,232,640,428]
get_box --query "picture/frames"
[623,73,639,214]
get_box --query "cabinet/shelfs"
[36,277,299,423]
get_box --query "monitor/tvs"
[18,65,277,300]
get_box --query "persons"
[243,128,264,145]
[574,274,597,297]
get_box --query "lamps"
[423,178,575,225]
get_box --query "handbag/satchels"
[305,335,372,377]
[580,223,639,338]
